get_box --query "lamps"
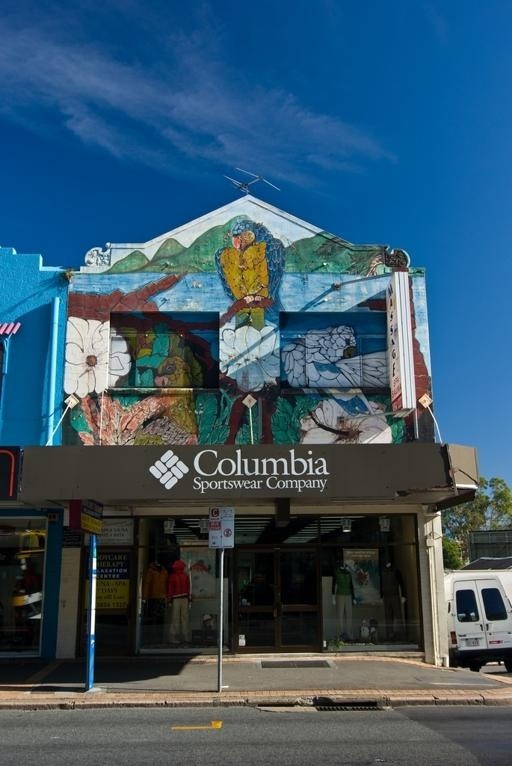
[341,519,352,533]
[164,522,175,532]
[379,519,390,532]
[201,520,209,533]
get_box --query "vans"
[440,569,511,676]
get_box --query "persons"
[380,562,408,640]
[142,557,193,644]
[332,566,357,641]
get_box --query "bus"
[1,531,44,639]
